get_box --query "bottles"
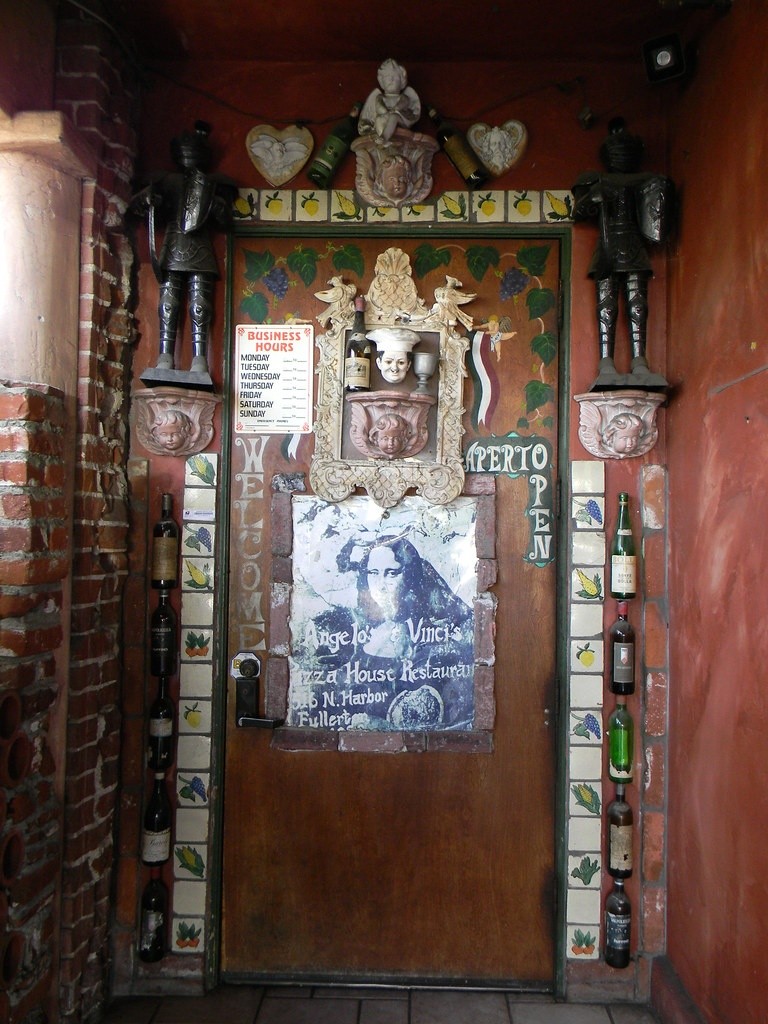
[428,106,492,191]
[607,696,634,784]
[603,879,632,969]
[609,492,637,598]
[607,783,634,878]
[151,491,181,589]
[139,865,169,962]
[151,590,177,677]
[345,297,371,391]
[307,102,363,191]
[609,600,636,695]
[148,677,175,771]
[140,772,173,864]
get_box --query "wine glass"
[413,351,440,396]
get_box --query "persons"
[129,124,235,374]
[373,59,409,140]
[368,414,412,453]
[568,121,673,376]
[366,327,421,383]
